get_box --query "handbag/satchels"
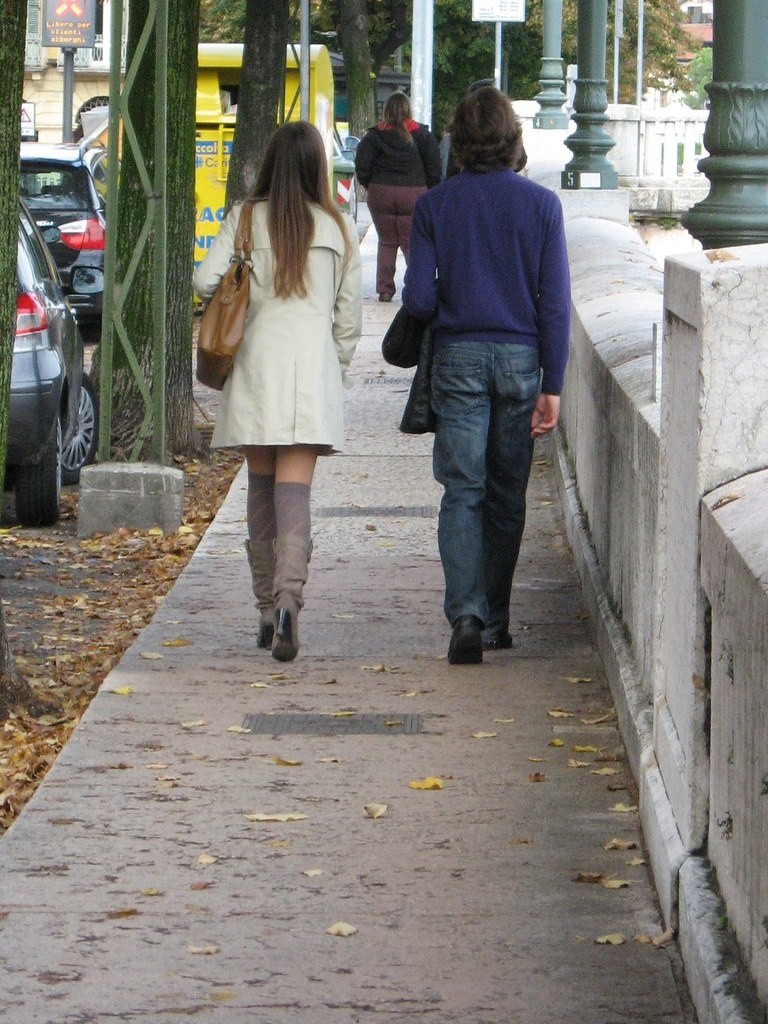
[195,202,256,391]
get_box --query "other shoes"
[378,292,391,302]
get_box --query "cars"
[10,195,100,527]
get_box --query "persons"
[355,92,442,303]
[401,86,571,666]
[436,77,529,183]
[192,120,365,661]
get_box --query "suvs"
[13,136,110,321]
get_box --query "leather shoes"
[447,614,483,664]
[481,633,513,651]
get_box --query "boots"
[245,538,278,650]
[272,536,313,662]
